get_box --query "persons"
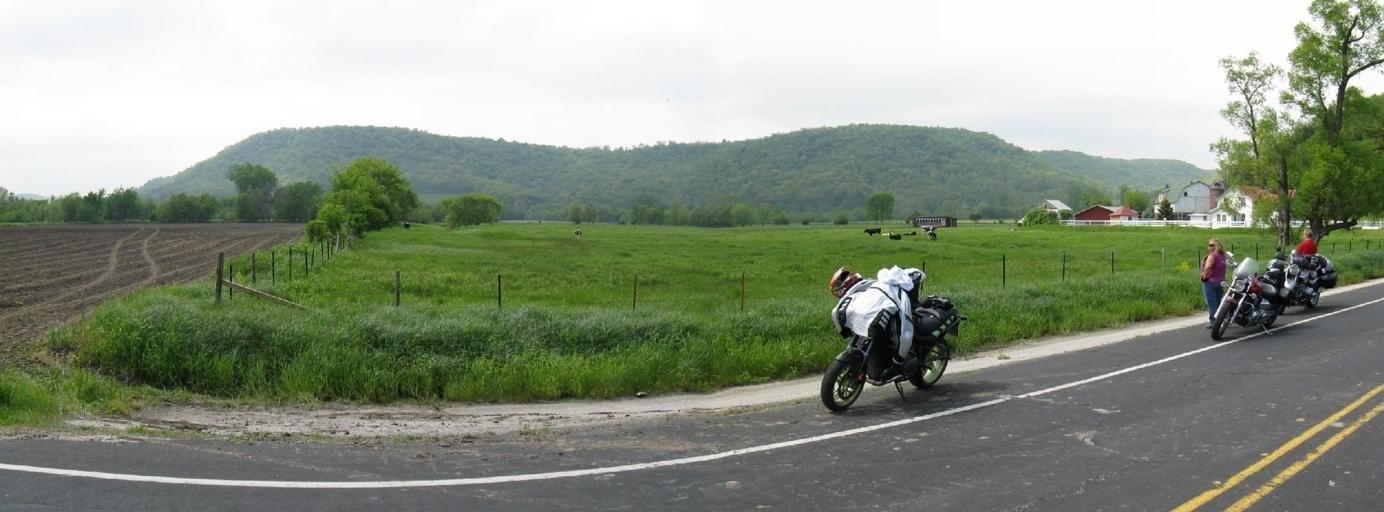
[1200,238,1227,330]
[1296,229,1318,254]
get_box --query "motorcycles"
[821,267,968,410]
[1211,246,1338,340]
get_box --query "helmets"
[826,265,863,298]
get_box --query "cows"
[889,232,901,240]
[863,227,881,236]
